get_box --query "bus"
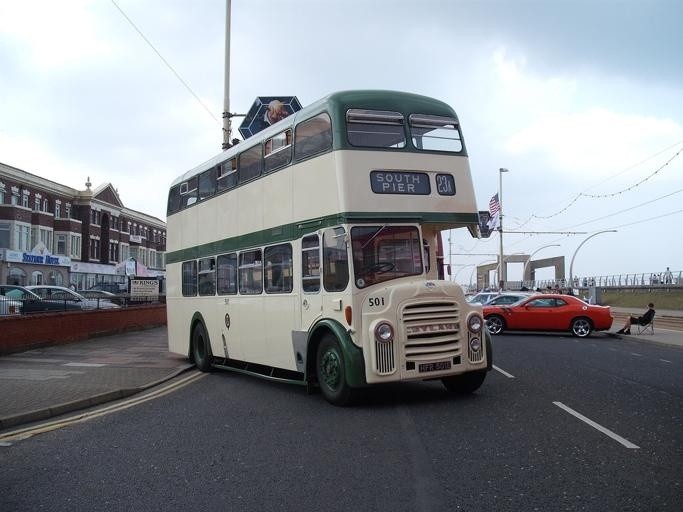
[166,90,492,405]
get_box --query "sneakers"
[616,329,631,335]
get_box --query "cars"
[465,292,614,338]
[0,282,127,315]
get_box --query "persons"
[518,284,574,296]
[662,268,674,283]
[616,303,656,335]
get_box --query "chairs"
[335,259,362,288]
[629,315,655,334]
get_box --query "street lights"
[499,168,509,292]
[523,244,561,283]
[452,259,496,285]
[569,229,618,289]
[493,251,525,289]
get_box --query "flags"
[485,192,500,231]
